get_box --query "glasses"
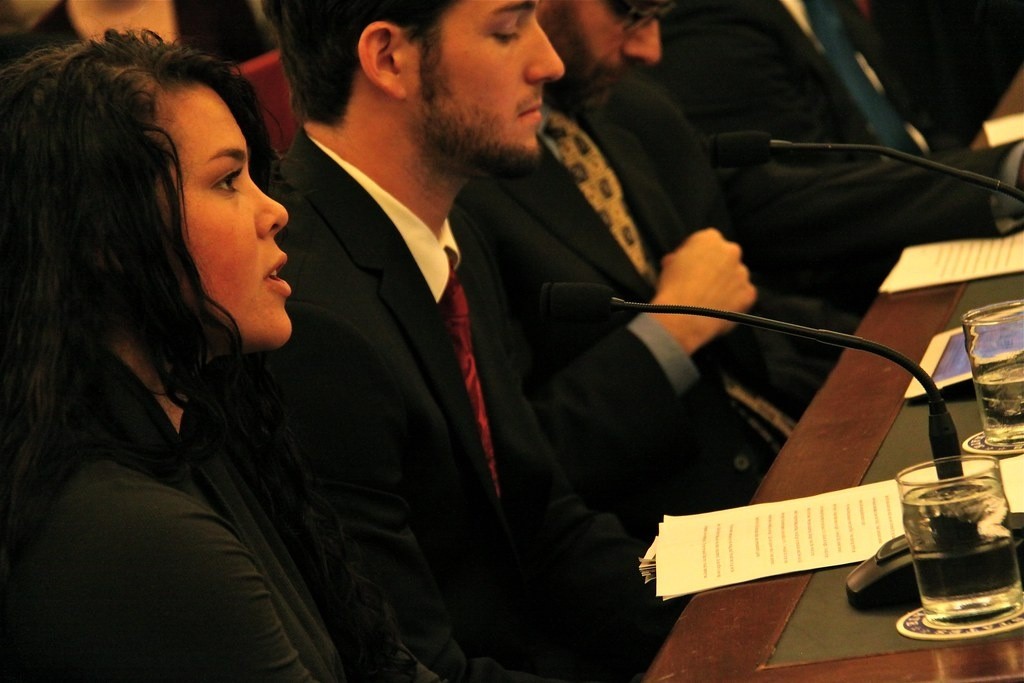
[610,0,677,32]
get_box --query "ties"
[544,110,653,286]
[802,1,925,162]
[445,247,500,502]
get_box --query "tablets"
[903,305,1024,399]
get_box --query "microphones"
[707,130,1024,203]
[538,280,1019,611]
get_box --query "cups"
[960,300,1023,444]
[894,452,1024,629]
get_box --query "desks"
[644,63,1023,683]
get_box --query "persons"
[206,0,683,683]
[1,22,364,683]
[452,0,804,569]
[14,0,275,94]
[678,0,1024,406]
